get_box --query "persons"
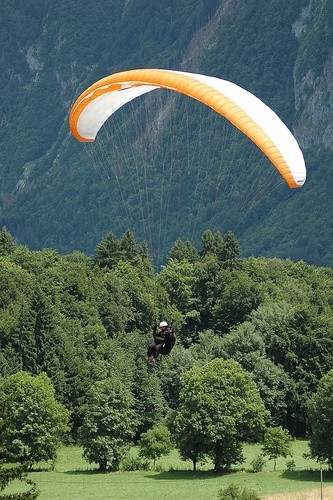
[147,321,176,359]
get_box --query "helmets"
[159,321,168,327]
